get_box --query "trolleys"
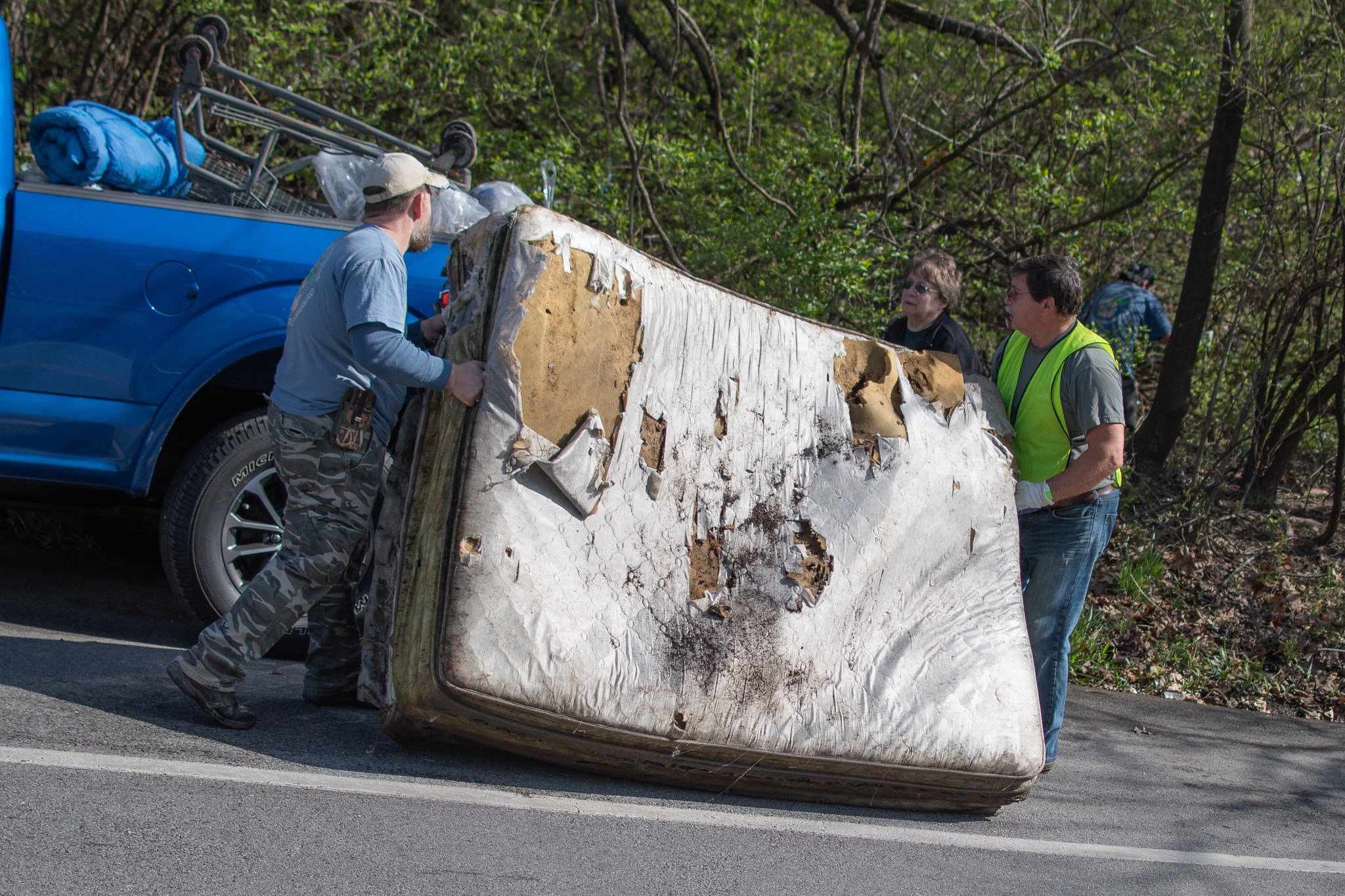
[149,13,481,221]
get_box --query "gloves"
[1014,480,1054,515]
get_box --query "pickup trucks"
[0,18,460,663]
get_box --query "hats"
[363,153,450,204]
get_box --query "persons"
[883,250,983,375]
[987,254,1125,773]
[1075,264,1173,433]
[166,152,486,729]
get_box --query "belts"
[1047,483,1117,508]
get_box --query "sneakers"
[167,661,256,729]
[302,684,375,709]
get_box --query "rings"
[476,400,481,402]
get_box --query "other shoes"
[1042,759,1056,771]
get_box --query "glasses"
[1006,289,1032,301]
[901,280,940,293]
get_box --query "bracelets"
[1044,482,1053,501]
